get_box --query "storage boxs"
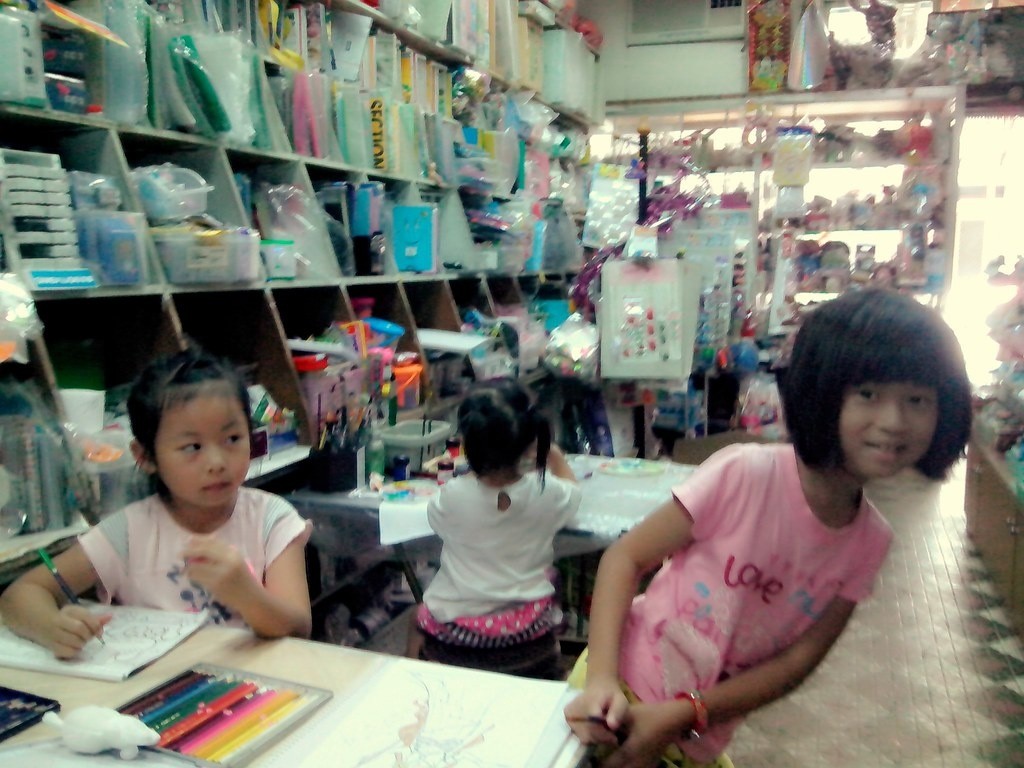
[75,212,147,285]
[378,422,452,470]
[42,40,87,75]
[68,171,120,215]
[262,240,296,281]
[530,300,568,333]
[137,167,214,222]
[391,364,423,410]
[0,6,46,107]
[155,227,259,285]
[380,0,606,124]
[479,244,524,273]
[43,73,90,112]
[362,317,404,354]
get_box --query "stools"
[417,626,563,680]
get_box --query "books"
[0,603,212,684]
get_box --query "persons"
[523,288,973,768]
[404,376,580,679]
[0,345,311,661]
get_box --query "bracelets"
[675,689,707,742]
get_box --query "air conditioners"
[626,0,746,47]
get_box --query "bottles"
[391,454,410,481]
[364,430,383,481]
[436,461,454,488]
[445,434,461,460]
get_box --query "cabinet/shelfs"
[964,393,1024,641]
[0,0,608,661]
[587,84,968,464]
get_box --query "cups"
[53,388,106,436]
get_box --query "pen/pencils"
[36,545,109,649]
[0,684,63,745]
[109,657,338,767]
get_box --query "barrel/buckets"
[392,363,423,409]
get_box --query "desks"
[291,452,699,673]
[0,627,593,768]
